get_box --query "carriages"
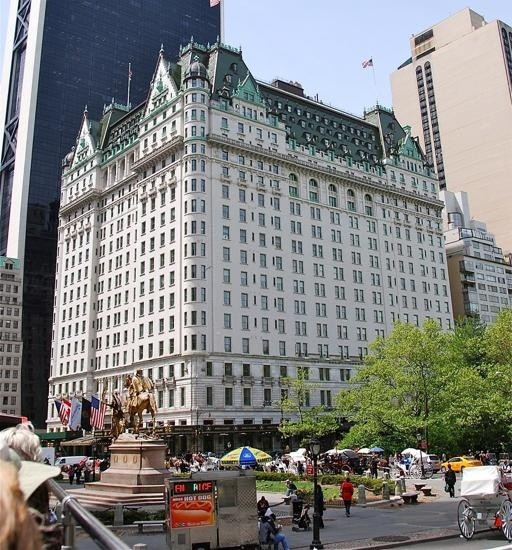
[360,447,435,479]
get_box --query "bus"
[486,453,509,464]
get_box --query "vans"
[54,455,88,471]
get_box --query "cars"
[87,456,98,466]
[439,457,483,474]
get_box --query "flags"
[90,396,106,429]
[68,396,82,431]
[81,396,91,431]
[54,399,62,413]
[362,56,373,69]
[56,398,71,423]
[210,0,220,8]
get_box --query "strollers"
[291,505,313,534]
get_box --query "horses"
[122,374,158,437]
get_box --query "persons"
[257,480,311,549]
[501,460,511,473]
[110,389,123,437]
[317,484,325,529]
[0,424,63,550]
[320,453,410,479]
[130,367,155,395]
[444,464,457,498]
[284,442,290,454]
[340,478,355,517]
[175,449,215,472]
[469,450,493,466]
[442,452,446,462]
[242,457,312,475]
[67,458,108,486]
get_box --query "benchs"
[261,542,279,550]
[415,483,427,490]
[133,520,166,533]
[400,493,419,503]
[282,495,291,504]
[421,487,432,496]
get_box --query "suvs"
[425,454,440,474]
[319,450,364,475]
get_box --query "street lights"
[306,438,325,549]
[416,433,427,480]
[194,404,203,455]
